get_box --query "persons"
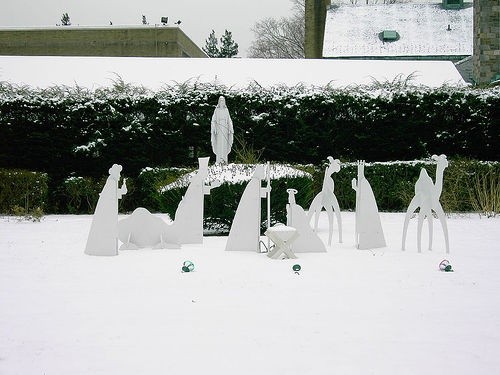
[210,95,235,165]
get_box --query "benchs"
[263,225,298,260]
